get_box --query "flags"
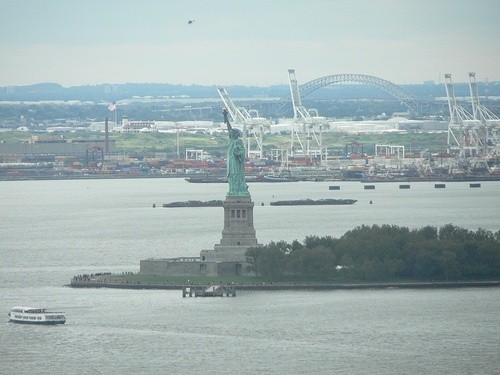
[107,100,116,111]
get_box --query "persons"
[223,109,250,196]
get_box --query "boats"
[187,161,499,183]
[9,306,65,324]
